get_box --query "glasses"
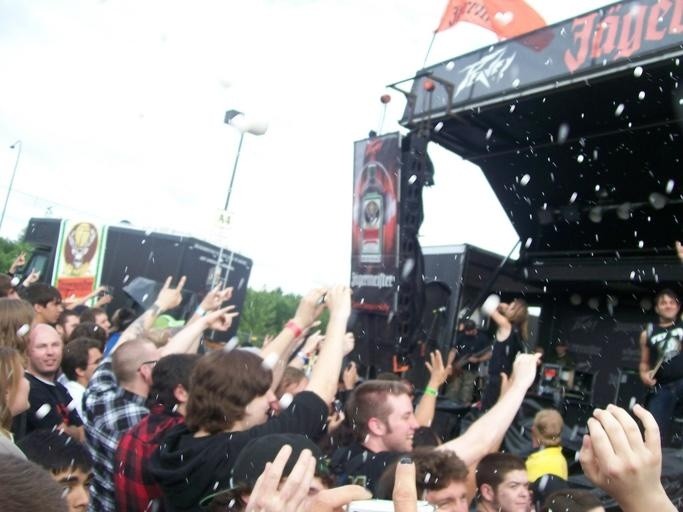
[137,361,156,372]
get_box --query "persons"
[639,242,683,451]
[373,452,471,511]
[15,431,95,512]
[330,349,540,495]
[553,338,572,369]
[1,253,237,353]
[203,433,373,511]
[2,347,68,510]
[61,342,111,418]
[84,276,238,512]
[114,355,200,510]
[540,402,676,511]
[10,321,84,453]
[334,358,360,449]
[444,319,493,405]
[525,407,569,499]
[484,294,529,415]
[147,286,353,511]
[275,363,345,453]
[474,453,528,512]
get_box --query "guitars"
[638,355,668,405]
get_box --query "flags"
[421,0,557,52]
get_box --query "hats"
[198,433,323,509]
